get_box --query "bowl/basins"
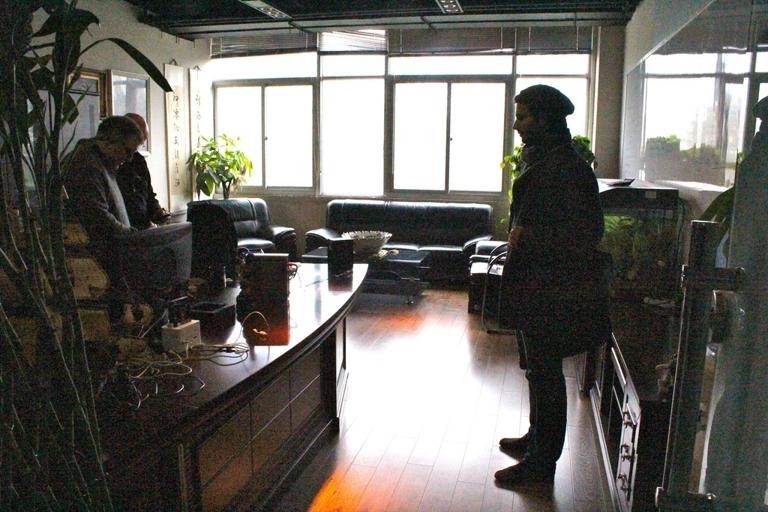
[342,231,392,256]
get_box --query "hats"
[521,84,574,115]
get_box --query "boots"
[495,371,567,485]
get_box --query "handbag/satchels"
[518,249,612,357]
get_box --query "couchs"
[304,197,494,286]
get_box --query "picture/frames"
[104,68,152,158]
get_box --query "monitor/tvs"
[112,222,193,302]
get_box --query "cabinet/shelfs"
[590,299,677,511]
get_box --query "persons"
[495,86,605,491]
[116,112,168,230]
[66,116,139,286]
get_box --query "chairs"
[186,198,298,281]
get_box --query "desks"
[105,262,370,512]
